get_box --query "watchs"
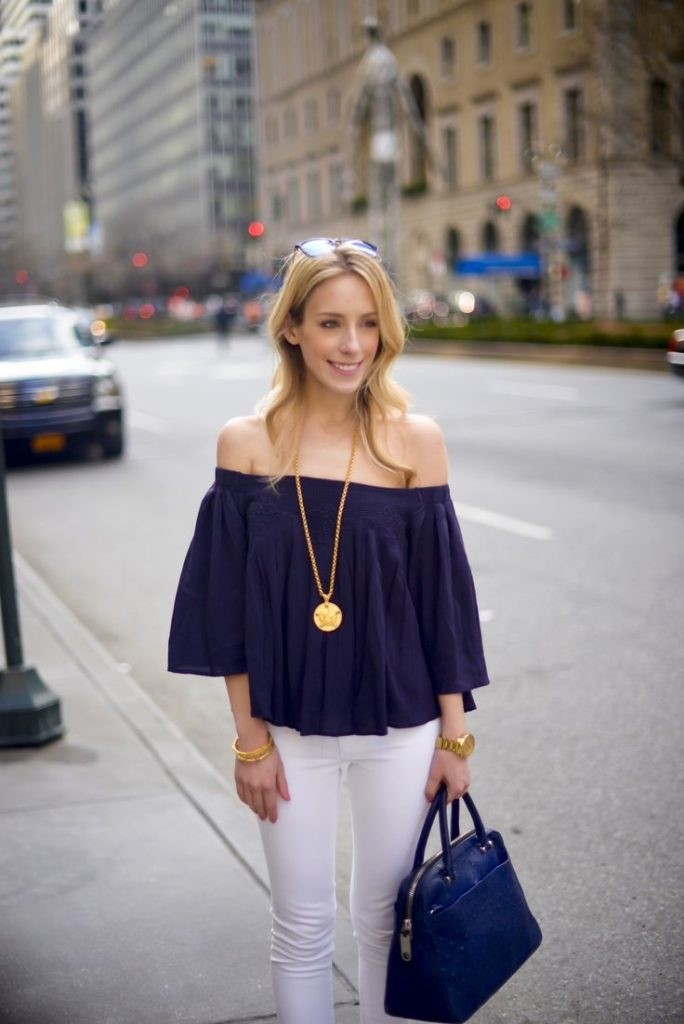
[436,734,476,758]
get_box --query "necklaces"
[291,398,360,632]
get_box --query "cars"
[0,304,123,466]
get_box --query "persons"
[167,238,542,1024]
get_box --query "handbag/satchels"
[384,776,543,1024]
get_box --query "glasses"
[292,237,377,266]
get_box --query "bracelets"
[232,735,275,764]
